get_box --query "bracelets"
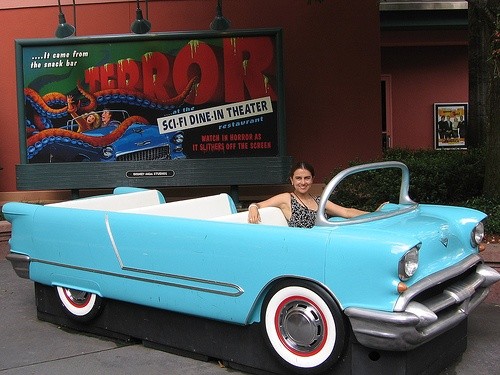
[248,204,259,210]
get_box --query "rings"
[249,218,252,221]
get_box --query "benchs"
[208,207,289,227]
[120,193,237,220]
[44,190,160,212]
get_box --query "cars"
[31,110,187,163]
[2,163,500,375]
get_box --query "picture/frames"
[434,102,469,150]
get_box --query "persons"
[438,115,465,139]
[248,160,371,228]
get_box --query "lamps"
[55,0,76,38]
[132,0,151,34]
[210,0,232,31]
